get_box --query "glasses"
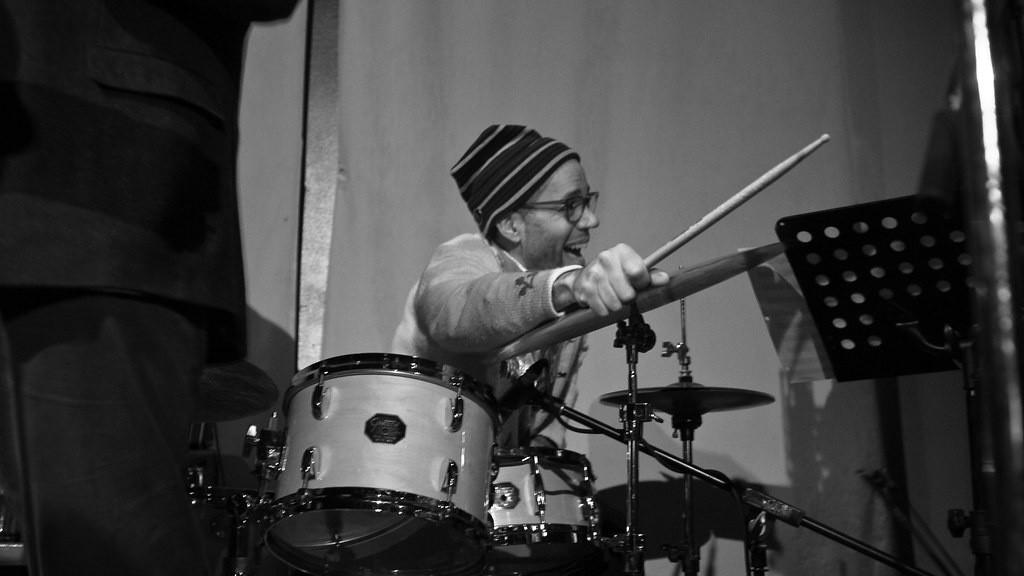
[519,190,599,223]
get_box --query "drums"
[254,353,503,576]
[484,447,603,576]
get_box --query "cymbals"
[492,242,785,361]
[597,382,776,417]
[190,359,279,422]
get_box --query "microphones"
[499,358,547,423]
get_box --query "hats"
[448,122,582,238]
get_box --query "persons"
[391,123,669,450]
[1,0,301,576]
